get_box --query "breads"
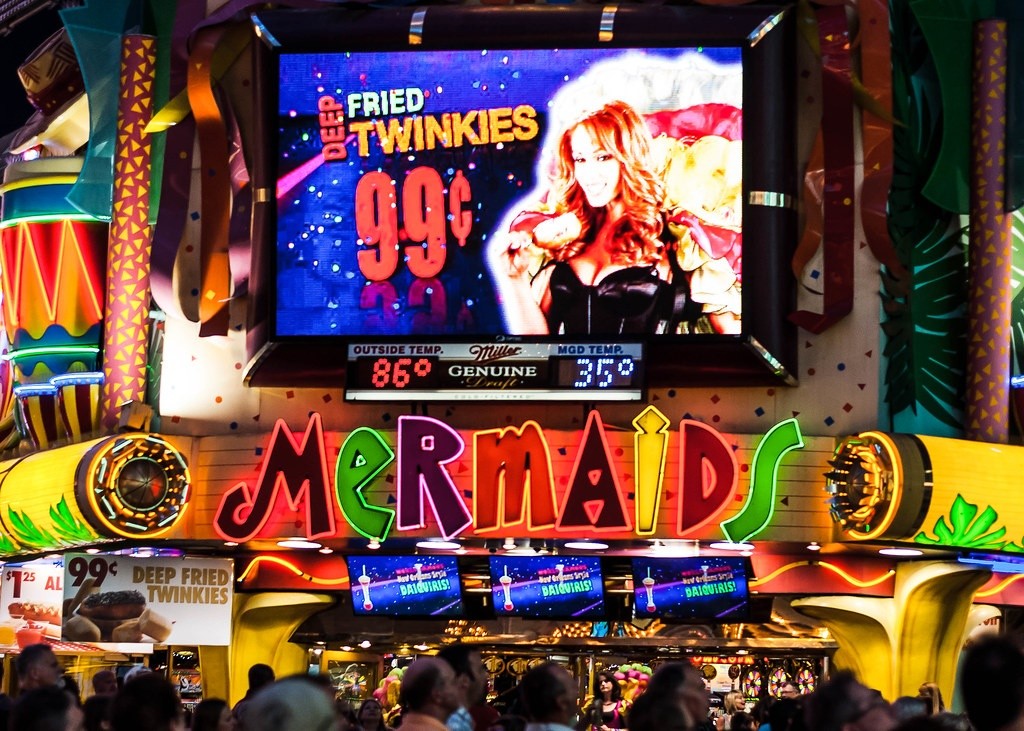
[62,578,174,644]
[8,601,62,625]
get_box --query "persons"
[0,638,1024,731]
[508,100,741,335]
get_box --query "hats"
[243,678,338,731]
